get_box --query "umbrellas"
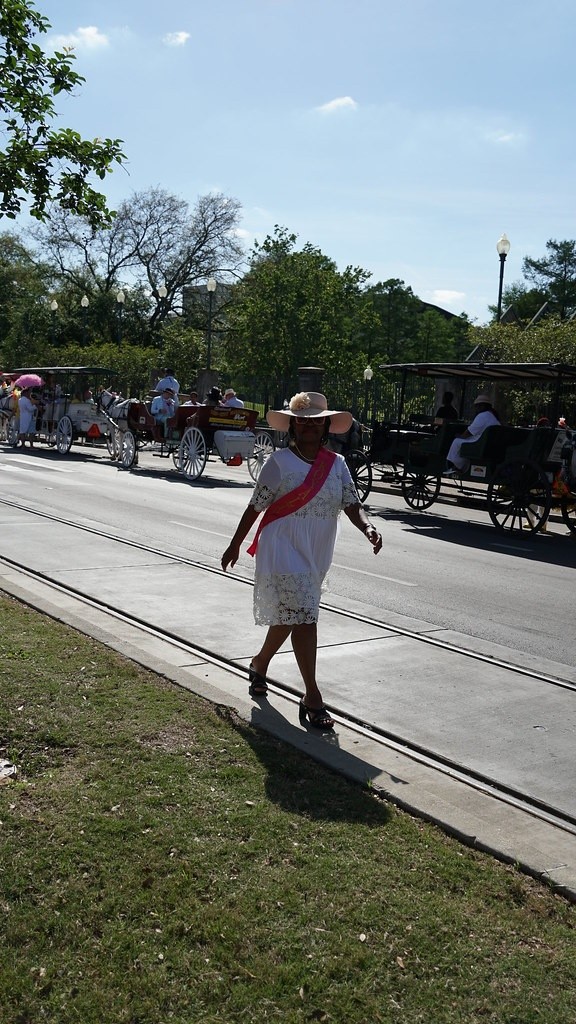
[16,374,46,386]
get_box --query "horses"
[0,381,21,441]
[94,385,139,464]
[277,399,361,453]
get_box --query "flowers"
[288,392,311,411]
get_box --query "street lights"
[117,290,126,349]
[363,363,374,424]
[494,232,512,325]
[50,299,59,346]
[206,277,218,369]
[80,295,90,347]
[158,284,168,321]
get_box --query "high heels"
[299,698,334,730]
[249,663,268,696]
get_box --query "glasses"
[290,416,326,426]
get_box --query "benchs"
[378,423,569,479]
[184,405,259,444]
[40,401,93,422]
[125,402,201,440]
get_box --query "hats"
[474,395,492,407]
[165,368,175,375]
[206,386,222,400]
[223,389,236,399]
[162,388,175,396]
[266,391,353,435]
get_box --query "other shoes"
[443,468,457,474]
[523,521,533,528]
[540,524,546,532]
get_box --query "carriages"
[0,364,122,456]
[324,360,576,541]
[94,384,276,482]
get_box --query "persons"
[155,368,180,406]
[436,391,458,419]
[523,418,549,533]
[224,389,244,408]
[71,390,94,404]
[18,389,37,450]
[151,387,175,437]
[221,392,382,730]
[202,387,224,406]
[184,392,201,405]
[443,395,502,474]
